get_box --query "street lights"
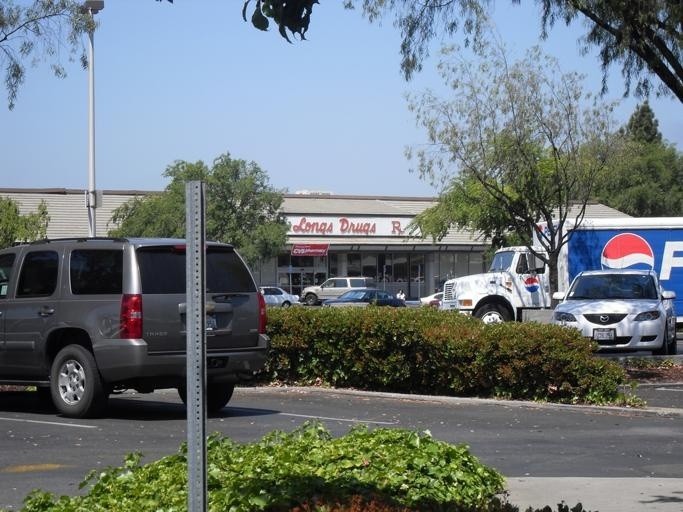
[76,0,106,239]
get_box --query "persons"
[396,289,406,300]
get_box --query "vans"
[0,235,272,420]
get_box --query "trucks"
[439,214,683,339]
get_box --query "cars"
[297,273,425,284]
[546,267,678,358]
[245,276,445,311]
